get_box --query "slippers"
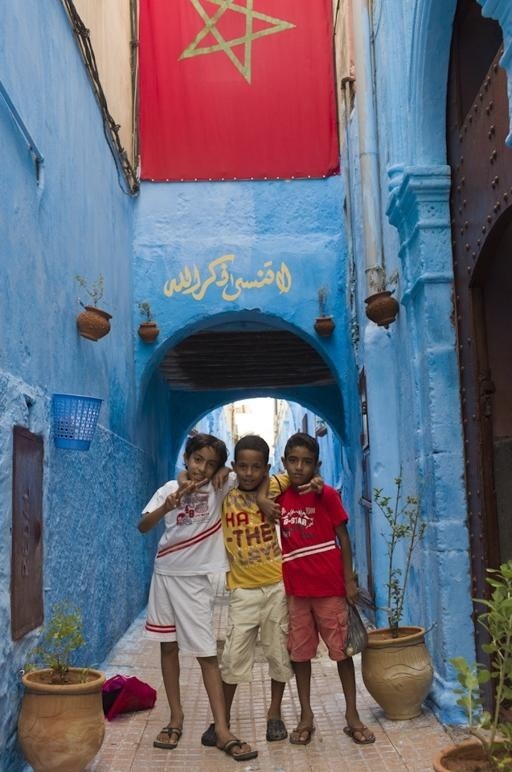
[154,728,181,748]
[266,719,287,741]
[202,723,257,761]
[343,724,375,744]
[290,727,316,745]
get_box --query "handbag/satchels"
[102,674,157,722]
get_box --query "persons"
[207,432,376,747]
[176,434,325,748]
[136,431,284,759]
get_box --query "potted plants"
[17,599,107,772]
[315,286,335,336]
[362,463,434,721]
[137,303,159,344]
[433,562,512,772]
[76,271,111,339]
[363,264,400,325]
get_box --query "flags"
[135,1,339,184]
